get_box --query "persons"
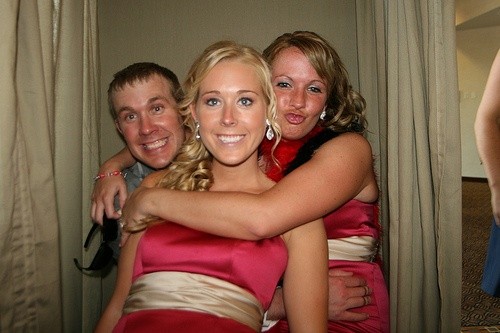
[74,62,373,333]
[91,29,390,333]
[92,40,329,333]
[474,45,500,298]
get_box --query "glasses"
[73,212,118,270]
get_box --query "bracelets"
[91,169,126,183]
